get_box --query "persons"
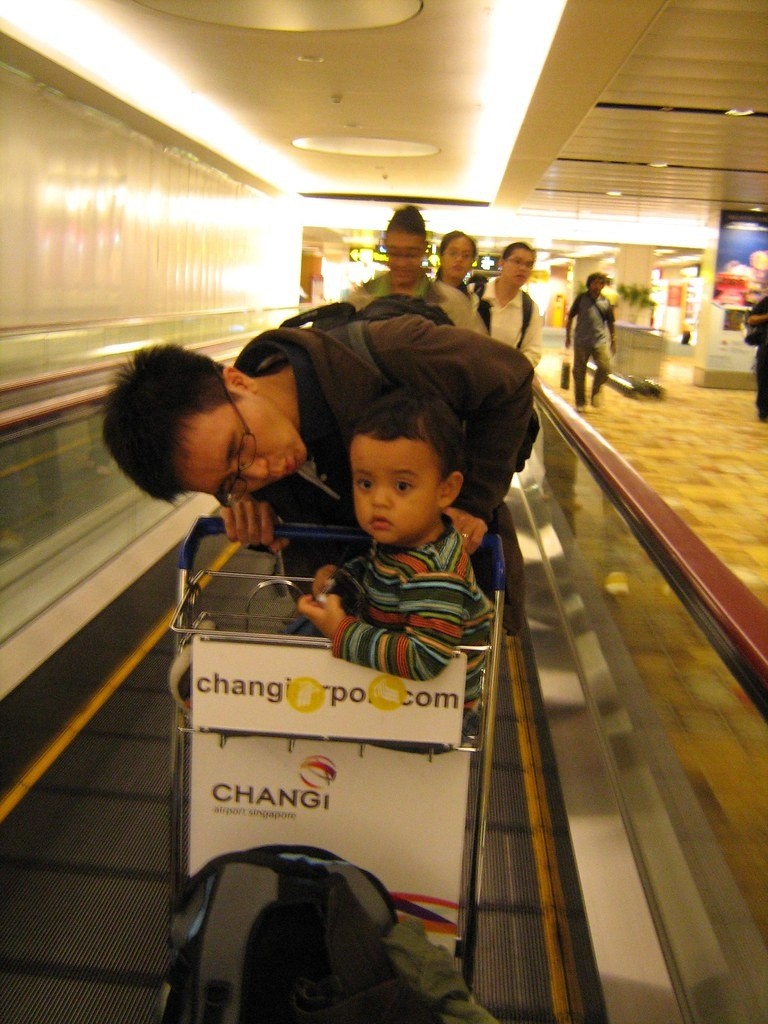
[747,295,768,420]
[565,273,617,413]
[169,384,495,754]
[95,316,534,638]
[340,205,545,372]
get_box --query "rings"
[461,533,469,537]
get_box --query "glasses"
[382,237,426,257]
[218,368,256,507]
[504,256,533,269]
[441,248,475,261]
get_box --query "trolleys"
[166,515,507,999]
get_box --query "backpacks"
[277,292,457,411]
[157,845,500,1024]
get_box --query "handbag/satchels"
[560,344,570,389]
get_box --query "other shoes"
[590,389,598,407]
[577,405,585,411]
